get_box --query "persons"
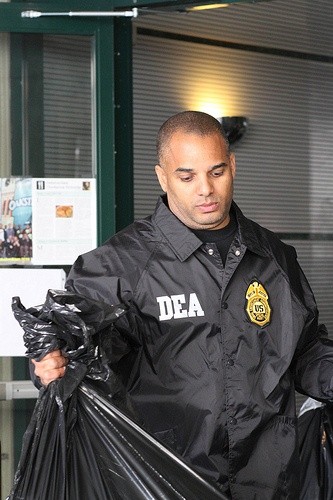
[30,110,333,500]
[0,222,32,258]
